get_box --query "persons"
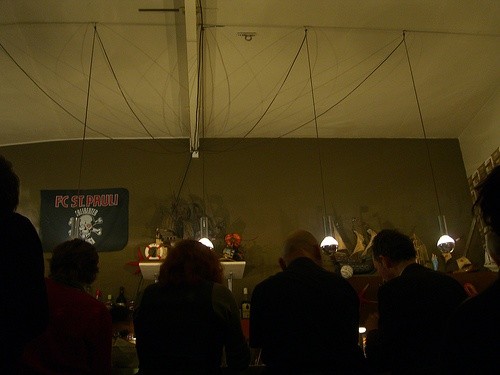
[24,238,112,375]
[0,156,45,375]
[443,166,500,375]
[249,231,368,375]
[133,239,251,374]
[365,229,469,374]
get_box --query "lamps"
[402,30,456,253]
[304,27,339,253]
[198,27,216,249]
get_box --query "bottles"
[116,286,127,322]
[105,294,113,312]
[95,289,103,301]
[240,287,251,320]
[86,286,91,294]
[152,273,159,284]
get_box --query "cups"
[359,330,367,354]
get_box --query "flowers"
[224,234,241,247]
